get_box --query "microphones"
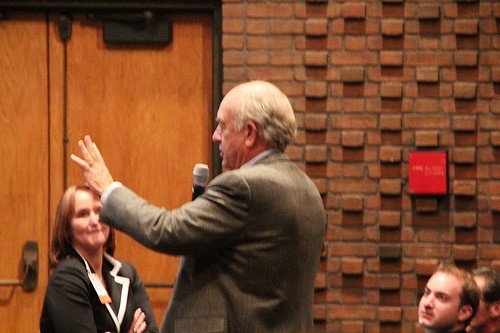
[192,164,210,201]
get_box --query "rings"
[89,160,96,166]
[133,327,136,333]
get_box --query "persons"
[40,183,159,333]
[466,267,500,333]
[70,79,326,333]
[418,264,479,333]
